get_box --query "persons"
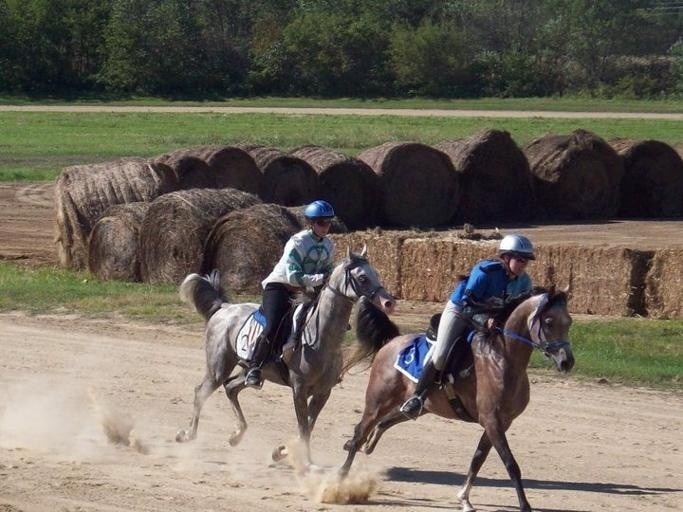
[398,233,537,421]
[242,200,339,387]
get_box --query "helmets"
[304,200,335,220]
[498,234,536,260]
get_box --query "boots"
[244,332,274,388]
[401,355,445,420]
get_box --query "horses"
[335,284,574,512]
[175,243,395,474]
